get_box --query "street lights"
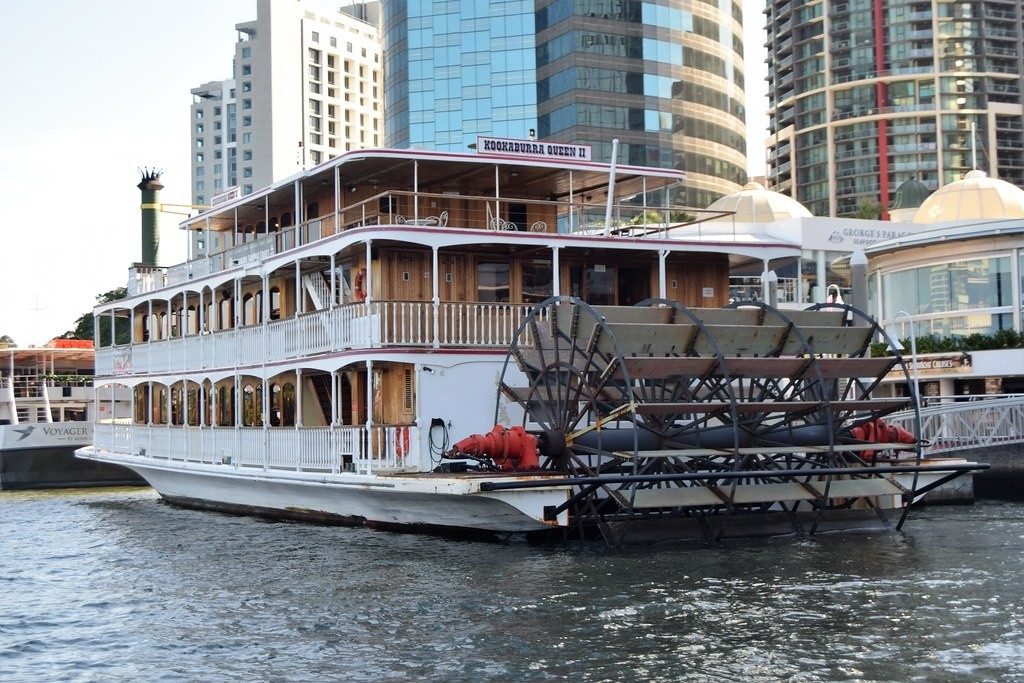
[885,311,924,458]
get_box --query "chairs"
[428,210,449,227]
[530,221,547,233]
[489,218,520,230]
[395,215,406,225]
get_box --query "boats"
[76,136,990,553]
[0,339,152,490]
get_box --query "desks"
[405,219,437,225]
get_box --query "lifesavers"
[395,422,409,457]
[355,268,367,300]
